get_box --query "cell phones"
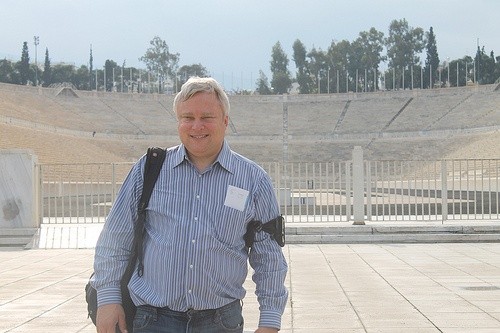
[278,216,285,247]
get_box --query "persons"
[90,76,289,333]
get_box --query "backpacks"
[85,146,167,333]
